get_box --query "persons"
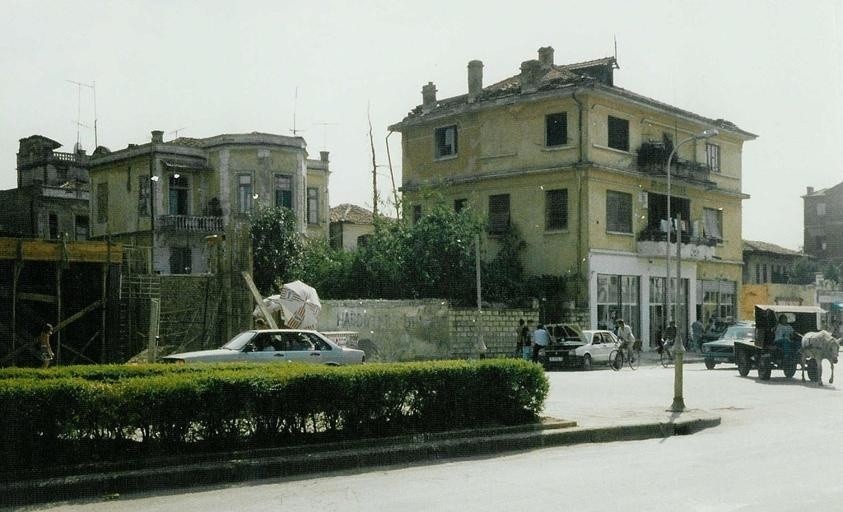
[522,319,534,359]
[770,314,804,369]
[615,318,635,363]
[692,317,705,353]
[516,318,524,353]
[712,313,724,329]
[39,323,55,369]
[661,320,676,364]
[533,324,549,360]
[286,336,302,350]
[261,336,276,351]
[705,318,716,335]
[655,325,665,354]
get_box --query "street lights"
[667,129,719,398]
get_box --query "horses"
[800,330,843,387]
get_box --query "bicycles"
[661,339,685,368]
[608,340,640,371]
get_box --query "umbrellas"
[254,279,321,329]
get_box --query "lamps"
[252,193,259,199]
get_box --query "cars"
[701,324,755,371]
[537,330,624,370]
[163,329,366,371]
